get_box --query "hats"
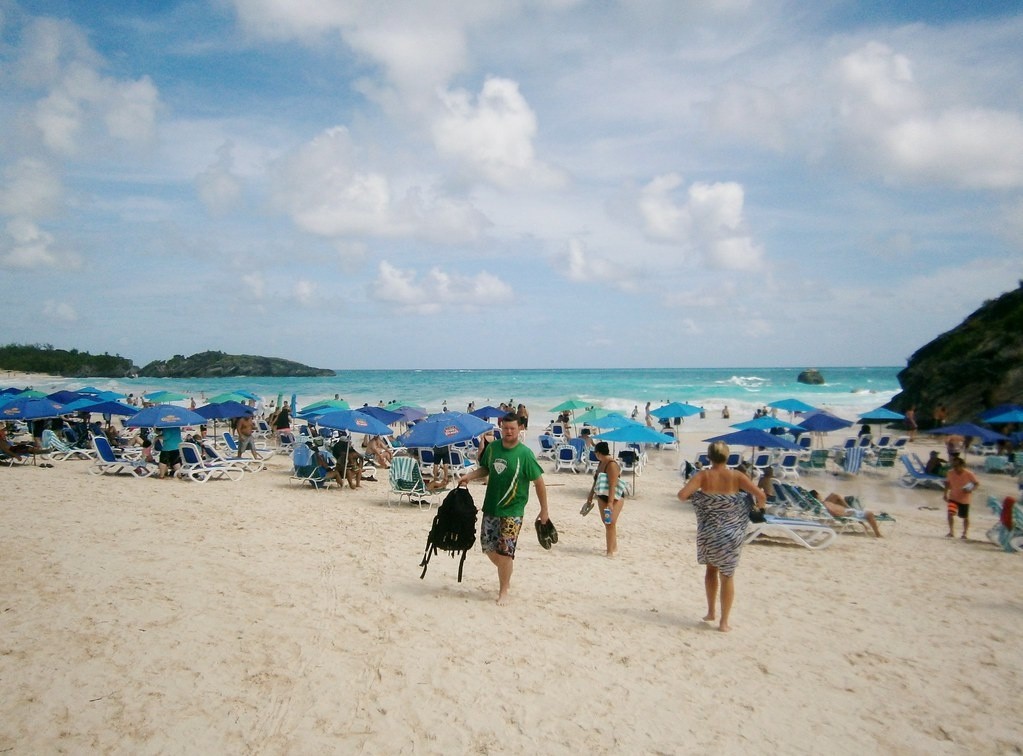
[930,451,939,455]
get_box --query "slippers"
[580,503,594,516]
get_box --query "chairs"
[0,420,1023,552]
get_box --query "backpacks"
[420,486,478,582]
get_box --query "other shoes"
[534,519,552,550]
[361,476,368,480]
[159,476,165,479]
[412,500,430,505]
[257,465,267,470]
[367,475,378,482]
[39,463,53,468]
[961,536,967,539]
[946,533,953,537]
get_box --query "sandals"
[546,519,559,544]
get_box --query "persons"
[0,430,52,461]
[943,457,979,539]
[586,442,625,558]
[721,406,729,418]
[335,448,362,489]
[457,412,549,606]
[365,435,393,468]
[236,410,257,459]
[276,409,292,433]
[906,405,918,442]
[678,441,766,631]
[312,446,343,487]
[478,431,497,486]
[432,445,448,481]
[155,427,182,480]
[645,402,653,426]
[805,490,884,538]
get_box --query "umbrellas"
[0,385,1023,490]
